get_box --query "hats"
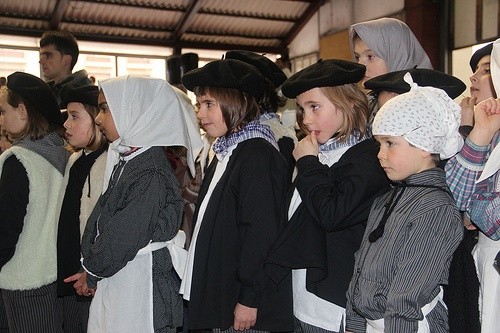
[225,49,287,91]
[470,43,493,74]
[282,58,366,99]
[59,85,98,107]
[363,64,468,100]
[7,72,63,130]
[182,59,272,98]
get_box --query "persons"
[344,71,465,333]
[444,36,500,333]
[349,16,435,124]
[224,50,299,183]
[0,125,13,154]
[177,59,293,333]
[38,29,96,122]
[263,57,389,333]
[0,71,64,333]
[442,42,498,333]
[54,84,111,333]
[362,63,468,110]
[65,73,204,333]
